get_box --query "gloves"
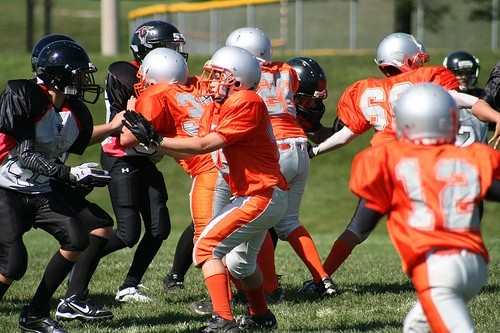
[331,116,344,132]
[68,162,111,187]
[122,109,163,149]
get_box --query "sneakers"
[56,289,114,324]
[191,300,213,315]
[264,288,285,305]
[237,305,277,333]
[17,306,68,333]
[115,283,152,303]
[293,277,338,303]
[163,272,184,292]
[232,290,250,305]
[191,311,241,333]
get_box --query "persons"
[0,21,500,333]
[350,83,500,333]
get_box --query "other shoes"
[303,279,315,288]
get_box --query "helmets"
[395,82,458,146]
[224,26,273,68]
[130,20,189,66]
[443,51,481,87]
[285,57,328,120]
[195,45,261,99]
[36,41,104,105]
[377,33,430,78]
[31,34,78,73]
[134,47,188,99]
